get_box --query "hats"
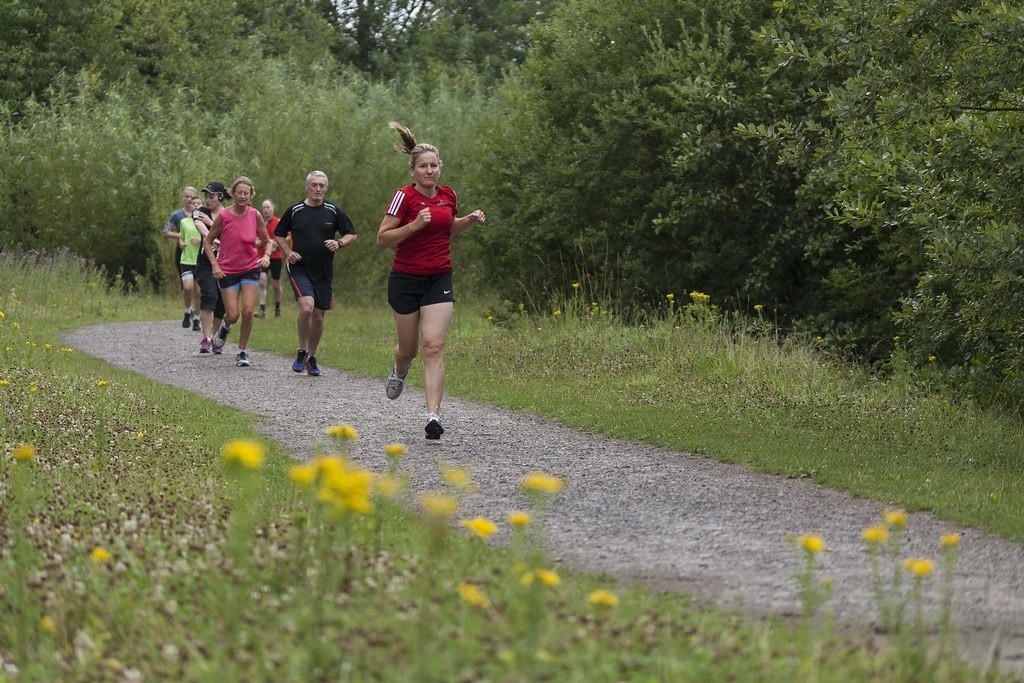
[201,182,225,199]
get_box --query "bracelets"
[266,253,271,258]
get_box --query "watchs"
[337,239,344,248]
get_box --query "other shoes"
[273,308,280,320]
[253,309,265,319]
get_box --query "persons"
[376,122,485,440]
[255,199,292,318]
[272,170,357,375]
[162,181,226,353]
[203,176,272,366]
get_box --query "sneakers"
[292,349,309,373]
[192,318,200,330]
[424,406,445,440]
[212,337,222,354]
[305,354,320,375]
[199,338,212,353]
[182,307,192,328]
[236,351,250,367]
[213,320,231,347]
[384,361,409,400]
